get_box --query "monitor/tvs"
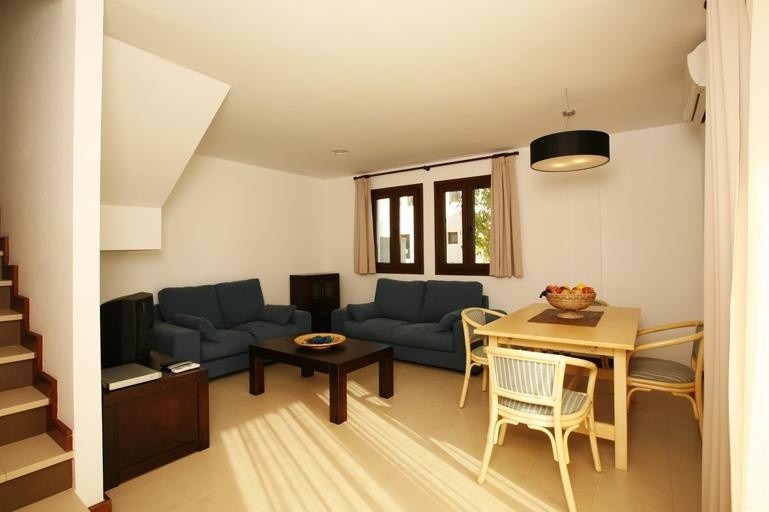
[100,292,153,368]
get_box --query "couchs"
[331,276,508,379]
[148,277,314,383]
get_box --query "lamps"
[530,108,610,172]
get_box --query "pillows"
[345,300,385,324]
[259,301,296,325]
[170,311,220,345]
[435,306,465,335]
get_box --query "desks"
[469,296,646,475]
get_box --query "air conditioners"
[683,39,708,130]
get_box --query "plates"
[294,332,346,352]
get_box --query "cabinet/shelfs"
[101,349,212,491]
[289,271,341,335]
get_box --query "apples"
[546,283,595,294]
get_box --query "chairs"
[609,318,706,446]
[476,344,600,512]
[457,307,515,410]
[590,298,614,371]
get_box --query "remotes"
[166,361,193,370]
[171,362,201,374]
[160,360,179,368]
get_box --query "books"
[102,361,164,393]
